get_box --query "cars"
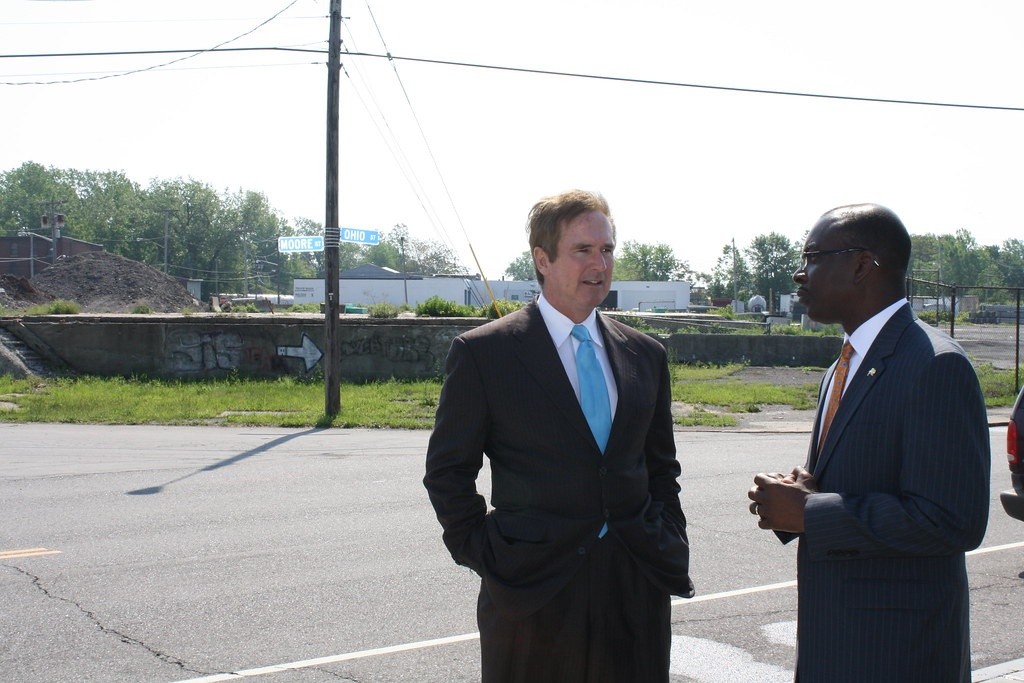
[223,297,272,315]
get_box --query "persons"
[423,191,696,683]
[747,203,991,683]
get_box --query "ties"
[570,324,612,539]
[816,339,854,461]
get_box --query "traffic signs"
[339,226,380,246]
[277,237,324,253]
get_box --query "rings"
[755,505,758,515]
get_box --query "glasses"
[801,247,880,271]
